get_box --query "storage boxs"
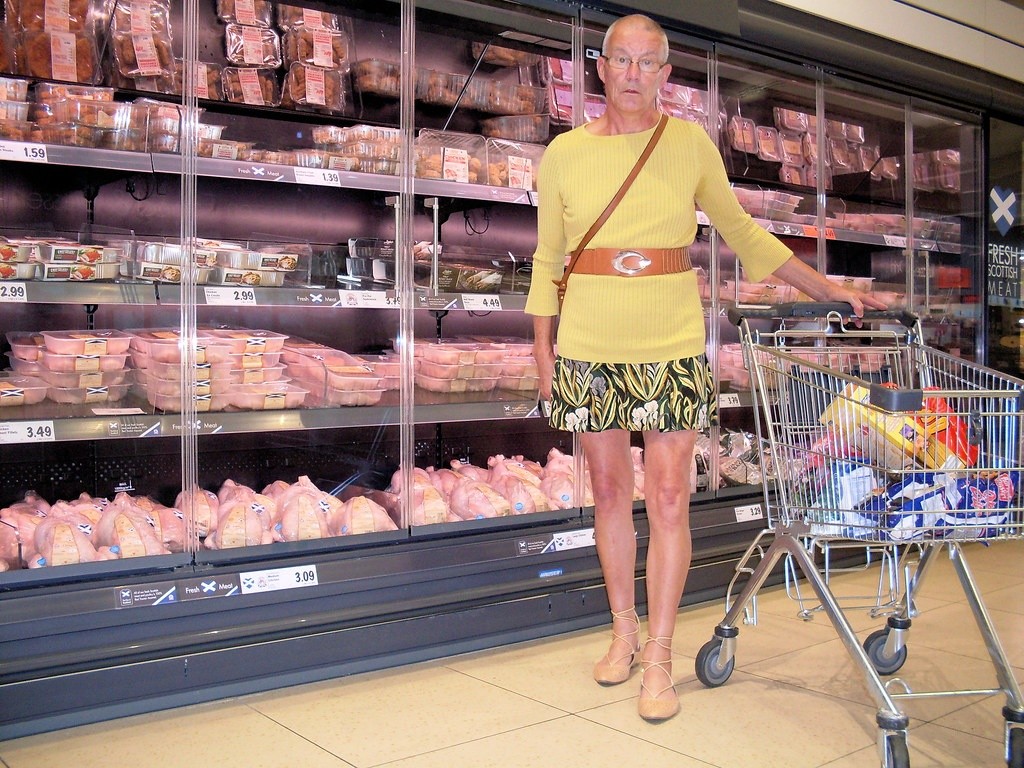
[818,375,967,472]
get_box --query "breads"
[0,0,544,190]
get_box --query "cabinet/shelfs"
[0,0,989,742]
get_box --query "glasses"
[602,55,668,73]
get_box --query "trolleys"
[696,304,1024,768]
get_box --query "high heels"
[638,635,680,719]
[592,605,641,687]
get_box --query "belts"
[569,246,694,277]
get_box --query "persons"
[525,15,889,721]
